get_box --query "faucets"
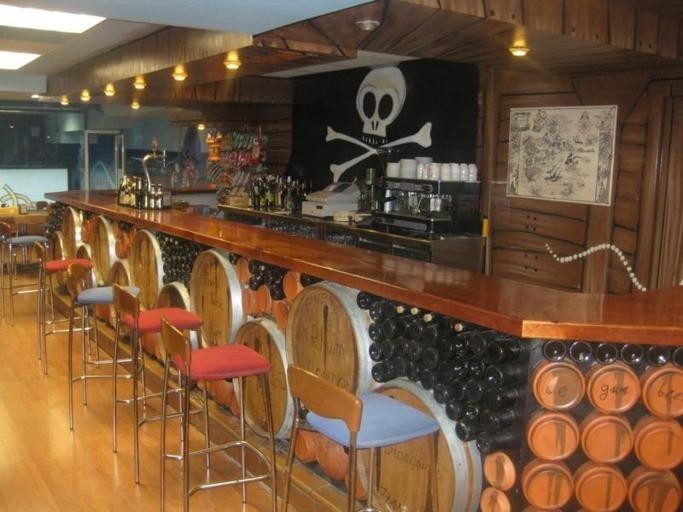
[142,149,168,191]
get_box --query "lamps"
[224,50,242,69]
[354,19,379,30]
[61,76,147,109]
[510,41,530,56]
[172,65,189,81]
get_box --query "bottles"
[355,291,533,454]
[155,231,213,296]
[116,174,163,210]
[250,173,313,212]
[247,259,287,301]
[228,252,240,265]
[539,339,683,369]
[382,187,453,216]
[298,272,323,288]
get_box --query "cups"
[384,157,479,183]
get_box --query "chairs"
[34,241,99,375]
[67,263,147,431]
[161,317,278,512]
[283,363,439,512]
[0,221,55,327]
[112,284,210,485]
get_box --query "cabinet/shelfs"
[0,209,48,266]
[224,210,483,276]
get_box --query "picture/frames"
[505,104,618,207]
